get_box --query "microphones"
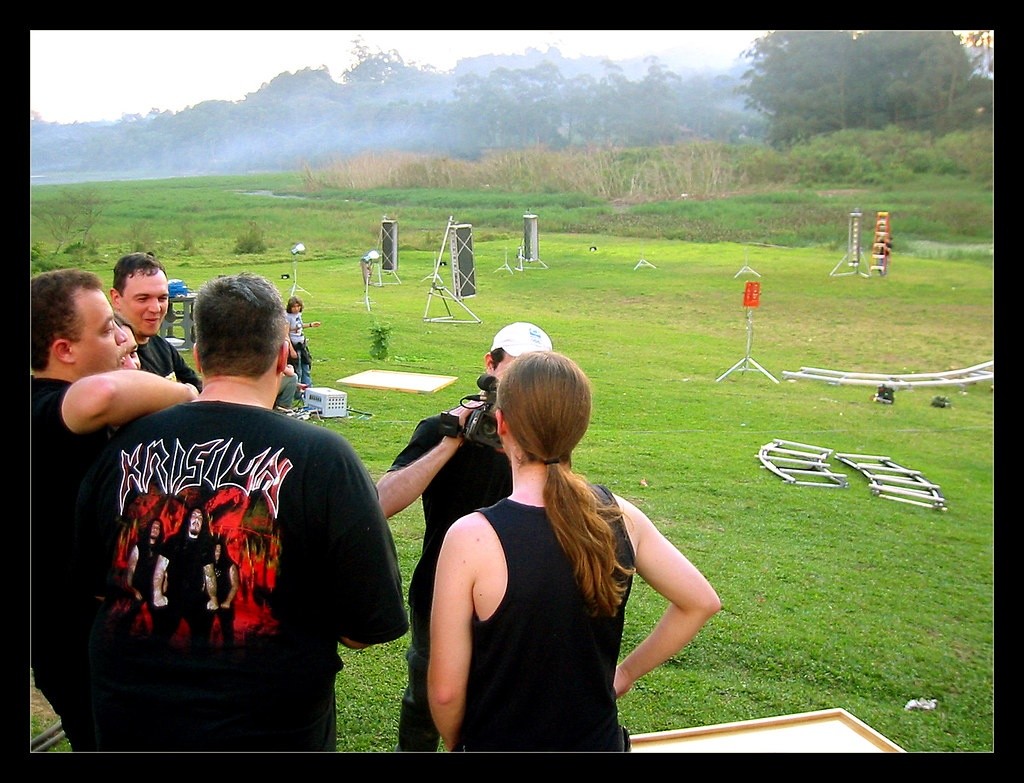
[477,374,500,391]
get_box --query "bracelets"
[310,323,311,327]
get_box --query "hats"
[490,322,552,357]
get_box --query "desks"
[631,707,908,753]
[160,293,198,351]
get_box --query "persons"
[375,321,721,752]
[31,252,410,752]
